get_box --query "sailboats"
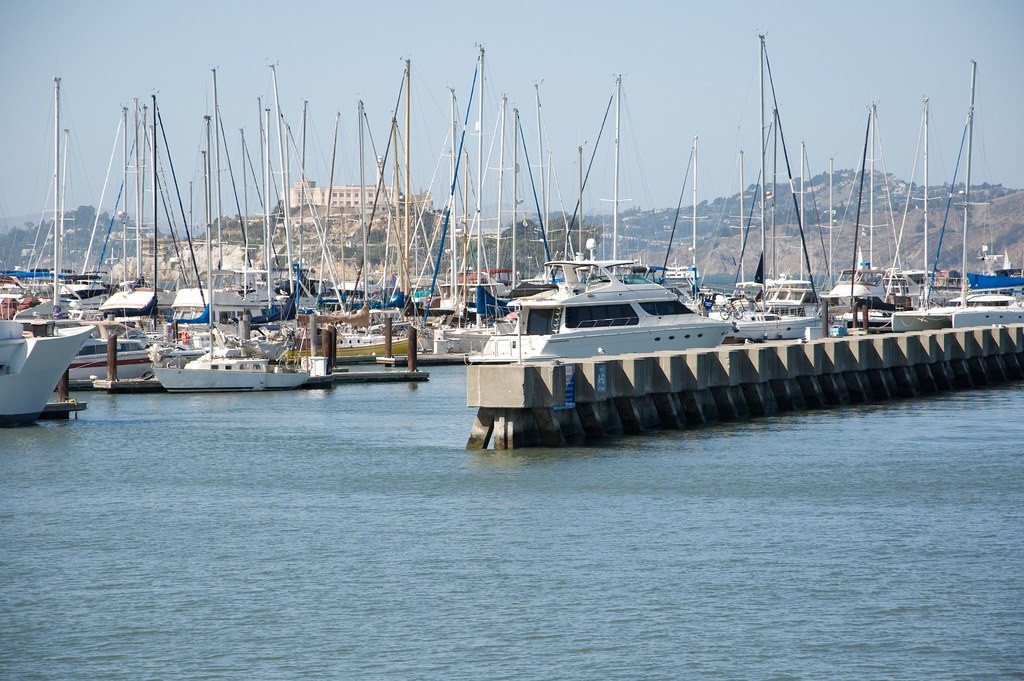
[0,29,1024,429]
